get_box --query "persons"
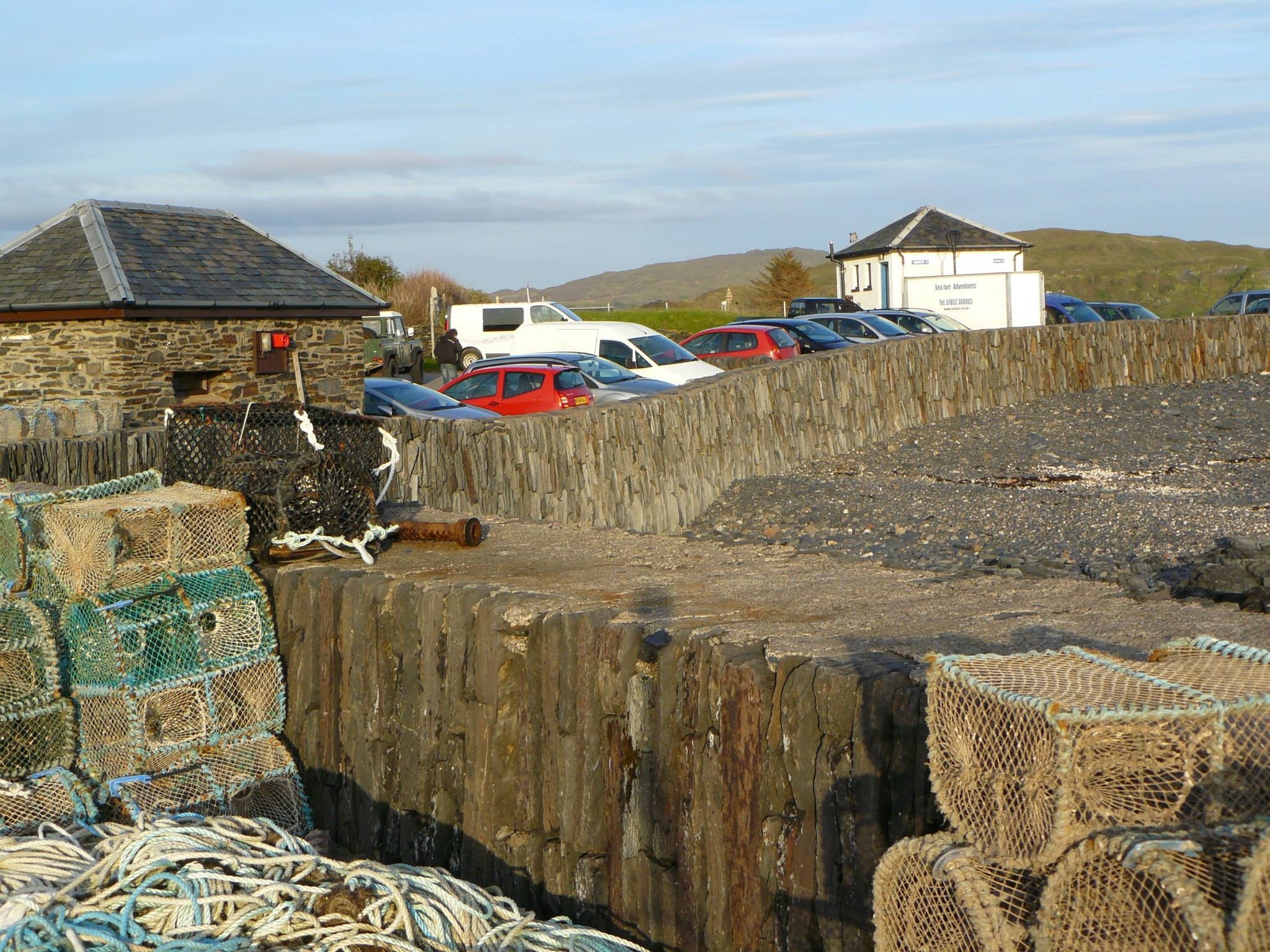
[433,328,465,386]
[840,295,863,313]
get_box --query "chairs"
[1102,310,1122,321]
[842,320,859,337]
[902,317,921,333]
[505,372,534,398]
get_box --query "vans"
[448,300,587,372]
[510,318,726,386]
[784,296,863,322]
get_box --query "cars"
[723,317,858,355]
[790,311,915,345]
[1084,301,1161,322]
[457,350,677,402]
[1206,287,1270,315]
[856,306,970,334]
[1044,291,1105,324]
[361,375,508,420]
[655,325,802,361]
[406,361,595,415]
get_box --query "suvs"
[358,310,427,388]
